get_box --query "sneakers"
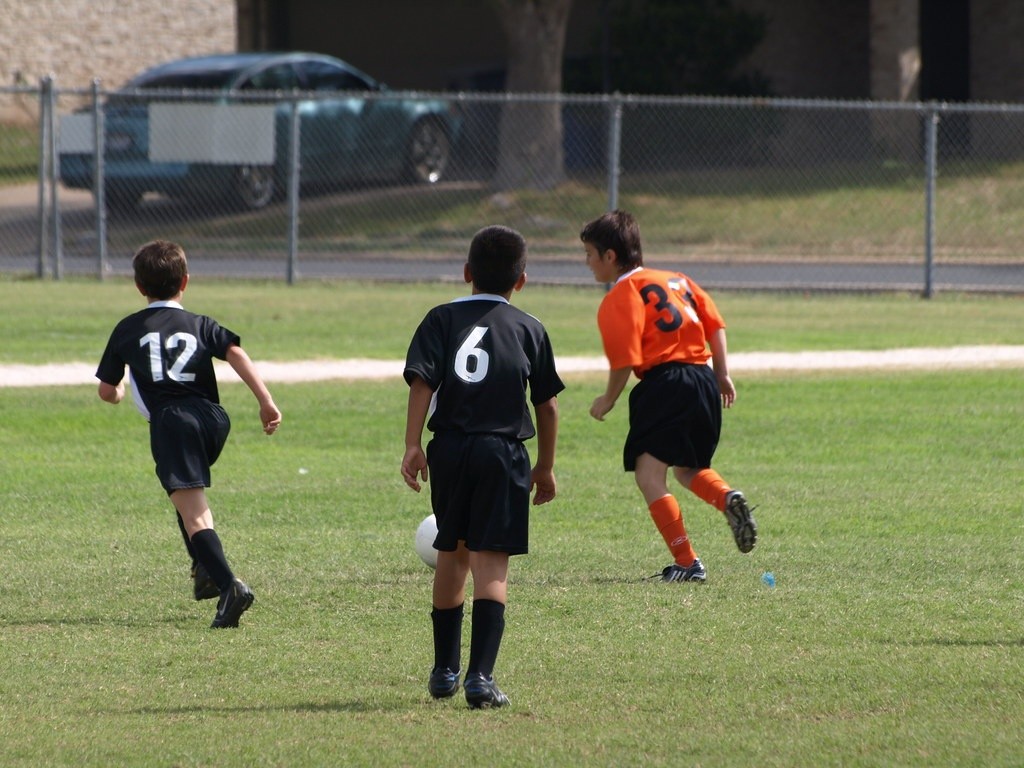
[209,577,255,630]
[643,557,708,584]
[190,562,220,601]
[428,666,463,699]
[722,490,761,553]
[463,669,513,709]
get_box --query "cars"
[60,51,462,210]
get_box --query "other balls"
[415,514,443,570]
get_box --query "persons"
[580,208,758,584]
[94,240,282,628]
[403,225,567,711]
